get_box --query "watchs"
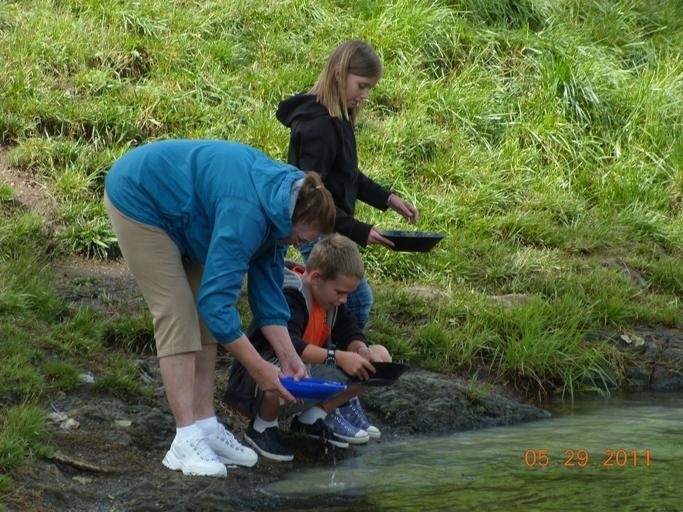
[323,348,336,369]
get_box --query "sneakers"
[199,422,258,467]
[289,394,381,448]
[161,435,227,478]
[244,418,295,462]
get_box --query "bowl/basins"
[379,231,446,251]
[334,362,412,386]
[277,377,347,403]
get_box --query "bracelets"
[353,347,372,356]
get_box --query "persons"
[275,39,418,446]
[218,232,384,463]
[102,138,338,480]
